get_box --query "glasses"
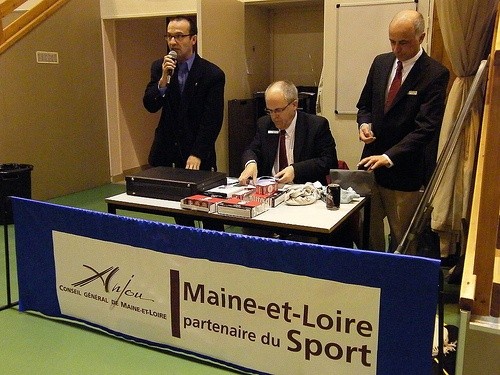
[265,99,296,115]
[164,33,191,41]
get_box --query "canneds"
[326,184,341,210]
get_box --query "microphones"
[168,51,177,76]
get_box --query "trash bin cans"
[0,162,34,225]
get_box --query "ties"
[279,130,288,179]
[179,63,188,97]
[384,61,403,115]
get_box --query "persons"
[143,15,225,172]
[238,81,338,185]
[356,9,451,256]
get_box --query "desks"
[105,177,372,251]
